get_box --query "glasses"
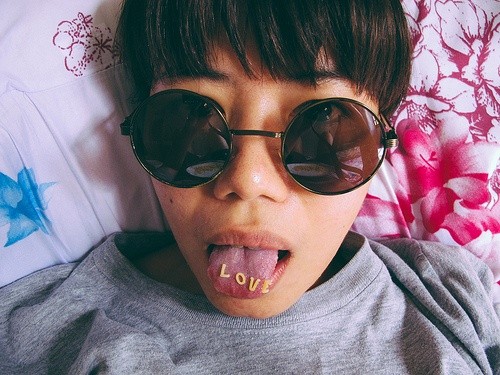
[119,89,400,196]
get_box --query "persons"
[0,0,500,375]
[178,133,230,179]
[285,131,333,179]
